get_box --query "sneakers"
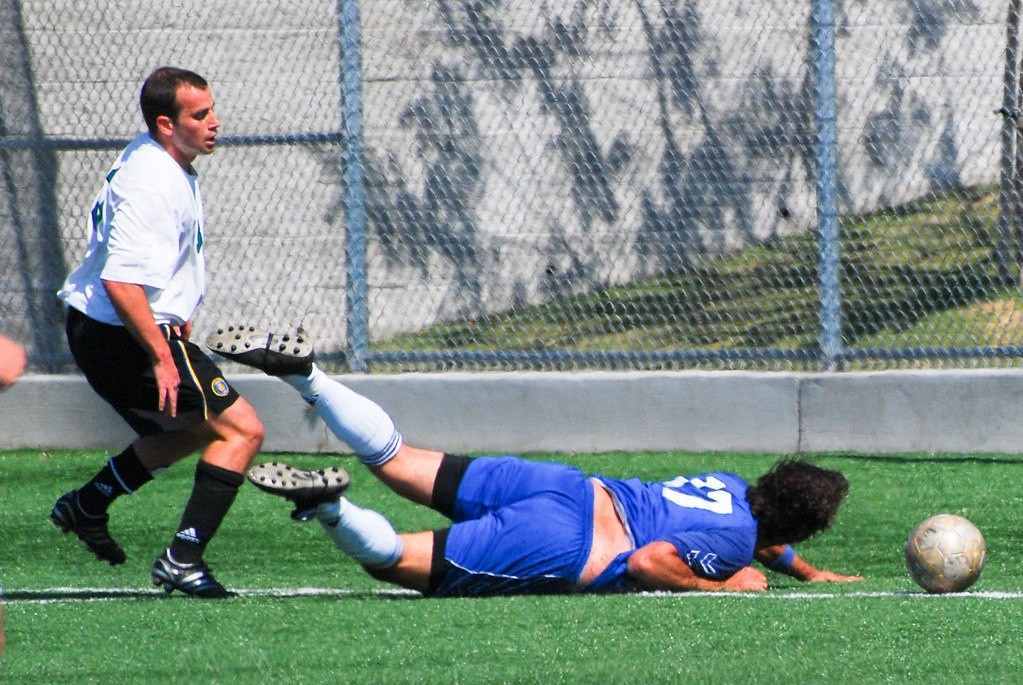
[245,461,351,521]
[47,489,127,566]
[205,326,314,378]
[152,548,240,599]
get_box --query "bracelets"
[766,544,795,572]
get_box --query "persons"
[47,66,266,599]
[206,323,866,594]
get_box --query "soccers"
[903,512,987,595]
